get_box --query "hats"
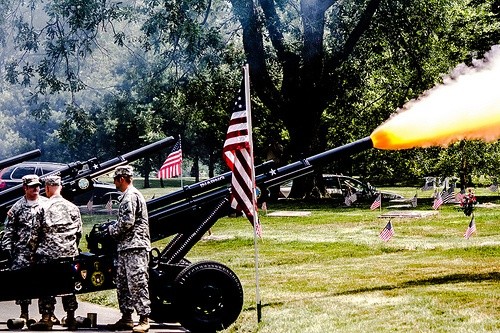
[22,174,42,186]
[112,166,133,177]
[45,176,60,187]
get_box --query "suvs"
[0,161,122,208]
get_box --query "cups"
[88,313,97,328]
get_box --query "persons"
[1,174,61,325]
[92,166,152,333]
[28,176,83,331]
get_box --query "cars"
[279,174,403,199]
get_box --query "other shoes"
[20,310,29,322]
[51,310,60,325]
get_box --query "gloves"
[98,221,116,231]
[6,249,13,265]
[94,228,110,242]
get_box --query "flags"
[431,185,478,210]
[157,139,181,178]
[465,215,476,240]
[411,195,418,207]
[344,188,357,206]
[222,75,265,244]
[369,194,381,211]
[379,221,396,243]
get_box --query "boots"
[67,309,78,330]
[132,314,150,333]
[30,313,53,331]
[107,313,134,331]
